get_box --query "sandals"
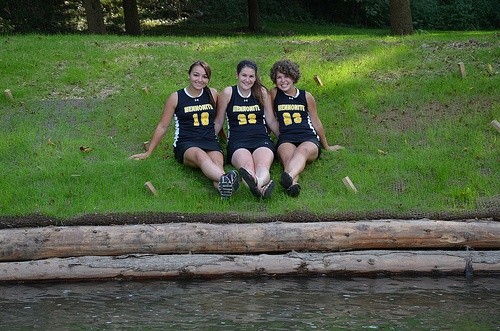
[260,180,273,200]
[238,167,261,197]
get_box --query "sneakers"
[218,176,232,197]
[225,170,239,193]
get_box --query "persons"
[128,60,240,199]
[213,59,281,198]
[265,59,341,198]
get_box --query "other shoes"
[279,172,293,189]
[287,184,301,197]
